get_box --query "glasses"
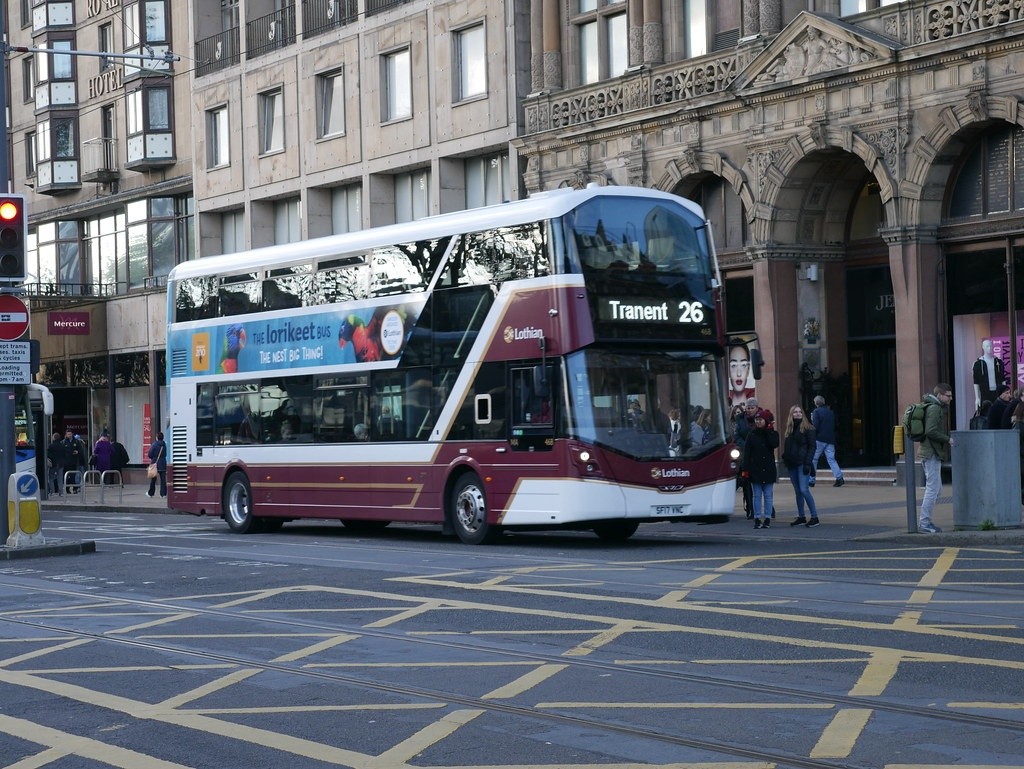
[942,393,952,397]
[729,361,750,370]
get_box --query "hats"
[755,409,769,423]
[746,397,759,407]
[996,385,1011,396]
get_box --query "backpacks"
[902,402,941,441]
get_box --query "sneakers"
[919,523,943,534]
[791,516,806,526]
[805,517,819,527]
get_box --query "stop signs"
[0,294,30,342]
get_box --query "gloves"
[803,464,811,475]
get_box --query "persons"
[728,337,754,404]
[970,386,1024,430]
[47,427,130,497]
[145,432,167,497]
[917,382,954,534]
[782,403,819,528]
[809,396,845,487]
[727,397,779,528]
[973,338,1006,411]
[197,354,714,444]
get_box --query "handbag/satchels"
[670,433,679,450]
[146,463,158,479]
[811,462,816,477]
[88,455,98,465]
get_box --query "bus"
[165,183,764,546]
[13,383,55,472]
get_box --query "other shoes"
[122,485,124,488]
[833,477,844,487]
[762,518,770,528]
[49,488,81,497]
[160,495,167,498]
[771,509,775,520]
[745,509,753,520]
[109,486,113,488]
[753,518,762,528]
[146,491,152,498]
[809,482,814,487]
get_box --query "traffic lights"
[0,194,28,282]
